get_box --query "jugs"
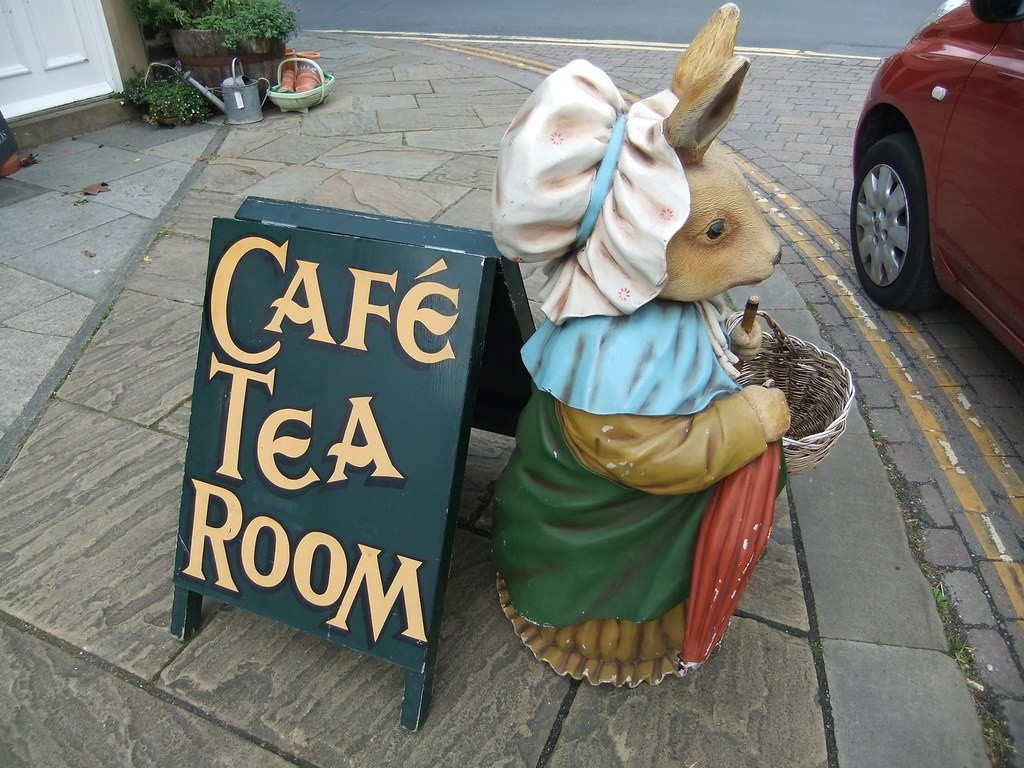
[179,58,270,127]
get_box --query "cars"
[849,1,1023,372]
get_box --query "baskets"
[729,310,856,474]
